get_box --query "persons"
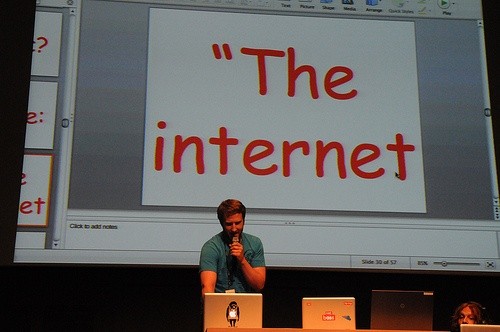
[198,199,266,301]
[453,302,487,326]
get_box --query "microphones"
[229,237,239,262]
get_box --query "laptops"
[460,325,500,332]
[371,290,433,331]
[302,297,356,330]
[204,292,261,332]
